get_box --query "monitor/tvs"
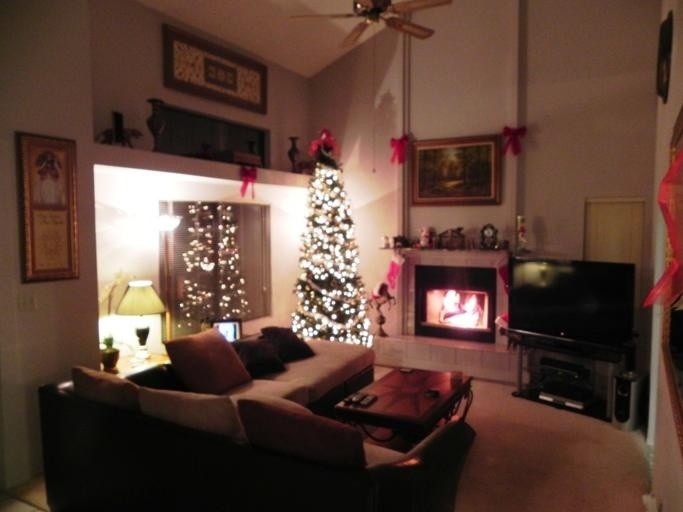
[211,318,242,343]
[507,256,635,364]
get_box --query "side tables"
[101,350,174,386]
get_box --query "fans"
[290,0,454,49]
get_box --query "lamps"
[115,280,166,358]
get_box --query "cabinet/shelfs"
[499,324,636,423]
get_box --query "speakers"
[611,367,648,432]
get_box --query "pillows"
[72,365,140,411]
[231,340,286,379]
[260,326,314,363]
[237,399,367,466]
[138,387,249,442]
[161,325,253,395]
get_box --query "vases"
[287,136,302,173]
[99,337,120,370]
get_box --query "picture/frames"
[160,23,269,113]
[411,135,503,207]
[17,132,81,283]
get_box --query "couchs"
[231,327,375,416]
[37,367,476,512]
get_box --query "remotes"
[344,392,377,406]
